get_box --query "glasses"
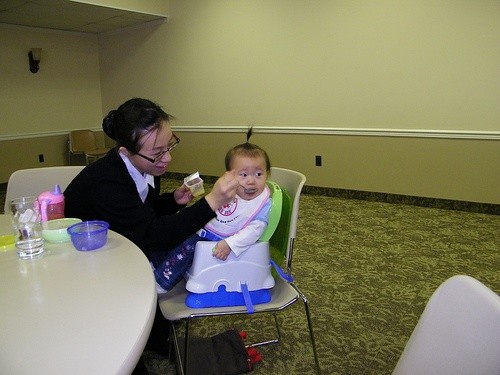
[128,132,180,163]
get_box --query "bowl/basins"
[41,217,83,244]
[67,220,110,251]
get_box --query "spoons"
[239,183,258,194]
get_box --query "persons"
[64,98,240,375]
[153,126,272,293]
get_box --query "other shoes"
[150,337,176,362]
[131,357,149,375]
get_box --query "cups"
[8,196,46,261]
[184,177,205,198]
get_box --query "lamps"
[28,47,42,73]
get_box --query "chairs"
[159,165,320,375]
[392,274,500,375]
[68,130,110,165]
[4,166,87,214]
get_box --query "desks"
[0,214,158,375]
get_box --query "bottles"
[37,183,66,222]
